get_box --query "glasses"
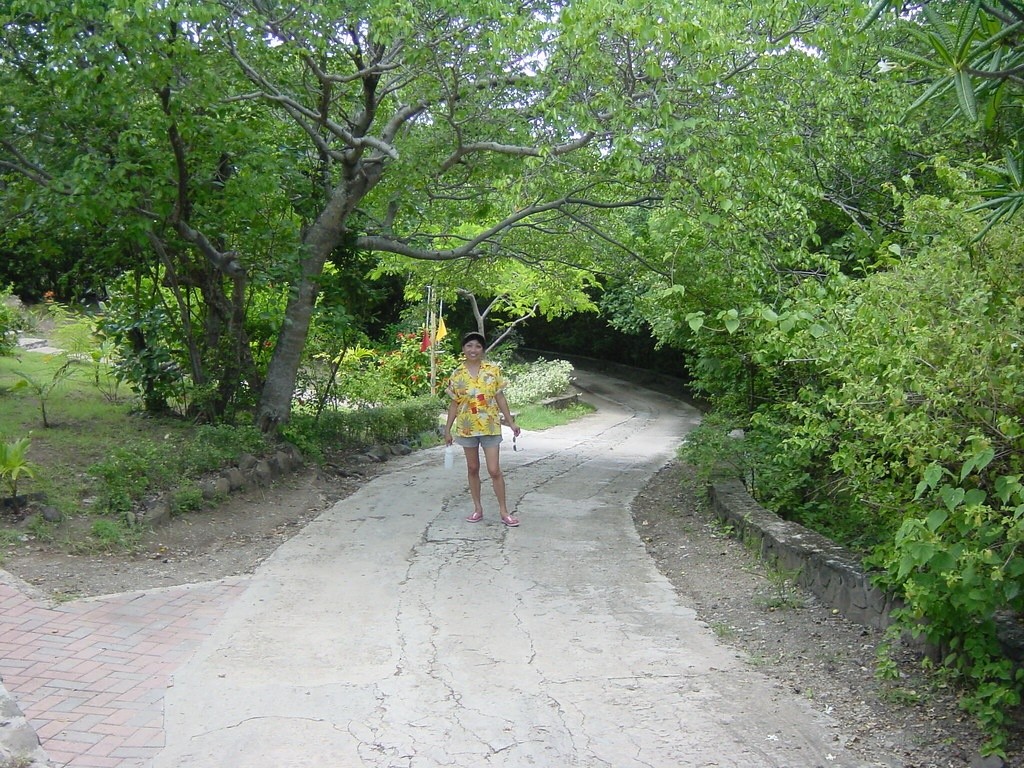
[513,436,524,452]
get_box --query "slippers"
[501,515,520,527]
[466,512,483,522]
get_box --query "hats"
[463,331,486,349]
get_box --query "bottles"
[444,442,454,470]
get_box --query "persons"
[445,332,520,527]
